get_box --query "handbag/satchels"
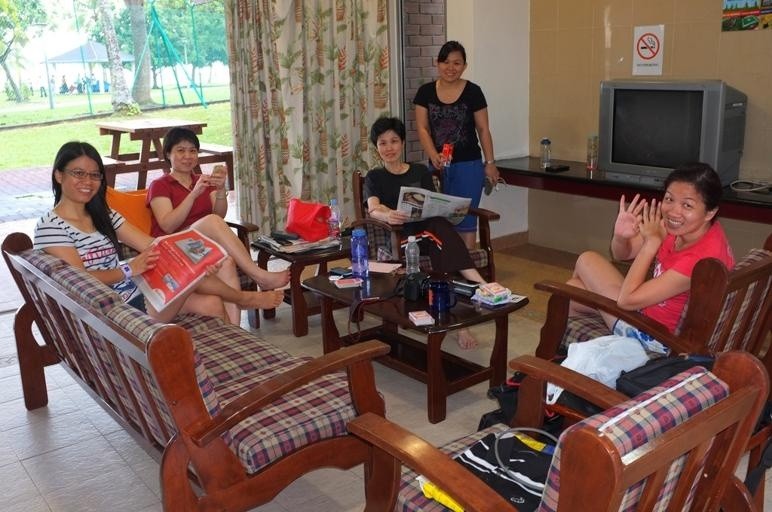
[285,199,331,242]
[478,364,562,435]
[617,353,714,398]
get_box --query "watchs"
[119,263,132,279]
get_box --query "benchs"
[100,155,125,188]
[198,142,236,191]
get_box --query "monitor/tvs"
[598,78,748,187]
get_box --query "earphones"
[495,184,499,191]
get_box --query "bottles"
[348,228,370,283]
[330,197,340,237]
[538,137,551,169]
[404,236,419,271]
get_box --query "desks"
[94,117,208,191]
[483,155,772,226]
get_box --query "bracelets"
[484,160,496,164]
[215,189,229,200]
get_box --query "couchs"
[2,232,392,512]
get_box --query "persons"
[362,118,487,349]
[145,128,292,327]
[413,41,500,250]
[33,141,285,325]
[566,163,735,354]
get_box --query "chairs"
[349,170,501,283]
[346,349,772,512]
[534,235,772,512]
[221,216,260,328]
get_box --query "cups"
[585,136,599,171]
[427,280,456,312]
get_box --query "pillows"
[104,185,151,237]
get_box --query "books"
[258,233,340,254]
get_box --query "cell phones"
[546,164,570,173]
[211,165,223,174]
[274,237,292,246]
[452,278,480,288]
[331,267,352,277]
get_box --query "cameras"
[404,272,434,301]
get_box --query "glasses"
[65,168,103,181]
